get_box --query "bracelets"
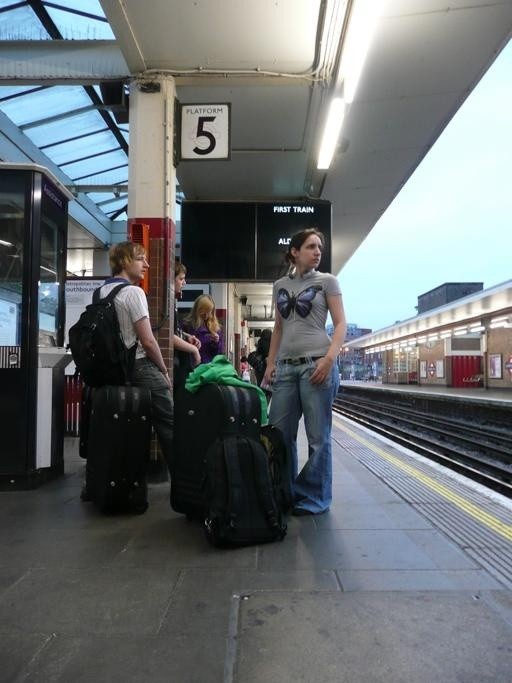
[161,369,168,374]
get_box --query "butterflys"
[278,285,322,320]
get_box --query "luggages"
[84,387,149,516]
[170,383,260,517]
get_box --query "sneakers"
[292,509,314,516]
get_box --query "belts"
[283,356,321,366]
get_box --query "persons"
[176,292,226,366]
[240,355,252,384]
[80,240,176,500]
[174,259,203,368]
[254,326,274,408]
[265,225,349,517]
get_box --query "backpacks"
[204,431,284,549]
[69,282,138,388]
